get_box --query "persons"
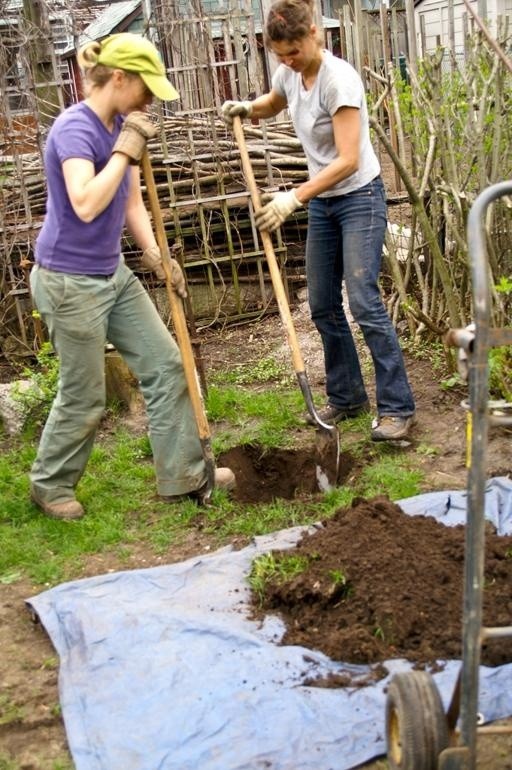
[215,0,419,445]
[22,28,240,523]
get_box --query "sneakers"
[304,397,371,425]
[29,485,85,520]
[370,408,419,441]
[160,466,238,505]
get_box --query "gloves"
[216,98,254,126]
[111,109,160,166]
[141,244,191,298]
[249,188,305,234]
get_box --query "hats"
[96,31,183,103]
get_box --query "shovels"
[141,146,239,507]
[231,115,341,493]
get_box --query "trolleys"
[387,180,512,770]
